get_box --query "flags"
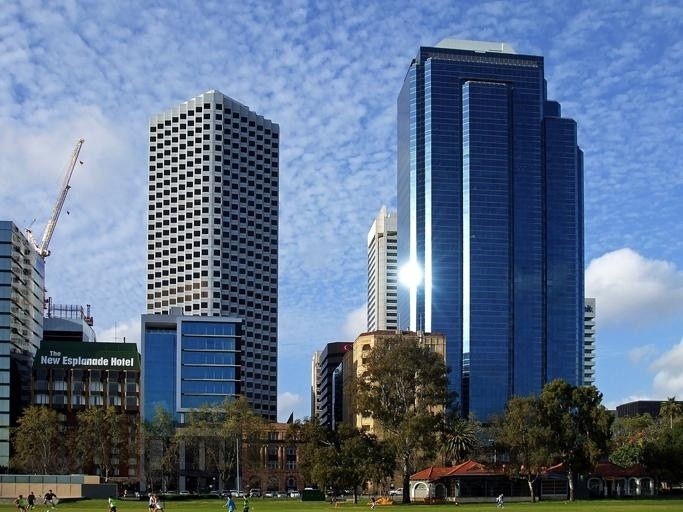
[286,413,292,425]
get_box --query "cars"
[147,490,190,497]
[135,492,144,499]
[209,489,301,498]
[388,487,403,496]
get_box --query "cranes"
[27,138,84,261]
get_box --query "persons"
[221,494,237,512]
[495,494,501,508]
[107,495,113,512]
[240,494,249,512]
[13,494,28,512]
[108,500,116,512]
[370,495,376,509]
[41,489,58,509]
[147,493,154,512]
[151,494,163,512]
[25,491,36,510]
[117,486,141,499]
[499,493,504,508]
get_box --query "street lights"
[488,439,497,463]
